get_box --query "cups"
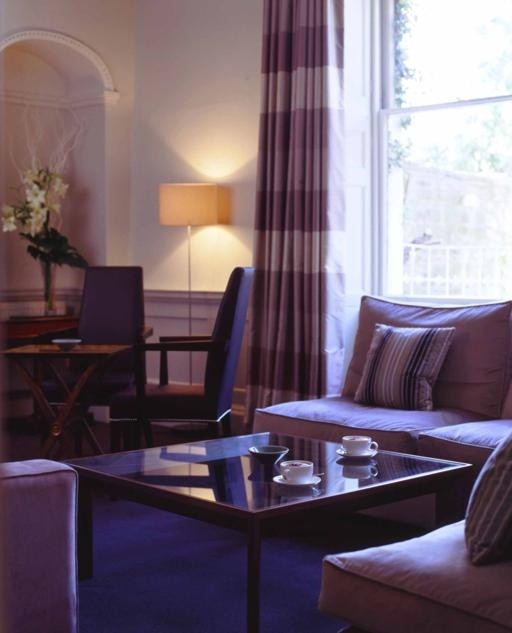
[342,467,378,479]
[280,460,314,481]
[341,435,378,453]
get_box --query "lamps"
[158,182,235,385]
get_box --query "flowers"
[0,106,92,299]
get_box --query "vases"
[40,261,55,317]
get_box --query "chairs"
[32,266,147,456]
[110,266,254,501]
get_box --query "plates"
[272,475,322,487]
[335,449,377,458]
[275,488,321,498]
[337,458,378,466]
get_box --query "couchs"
[318,518,512,631]
[0,459,80,633]
[254,295,512,532]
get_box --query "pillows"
[464,432,512,569]
[353,324,456,410]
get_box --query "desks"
[0,315,79,435]
[61,432,474,633]
[1,343,131,458]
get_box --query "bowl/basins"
[247,465,280,483]
[248,445,289,463]
[52,339,82,350]
